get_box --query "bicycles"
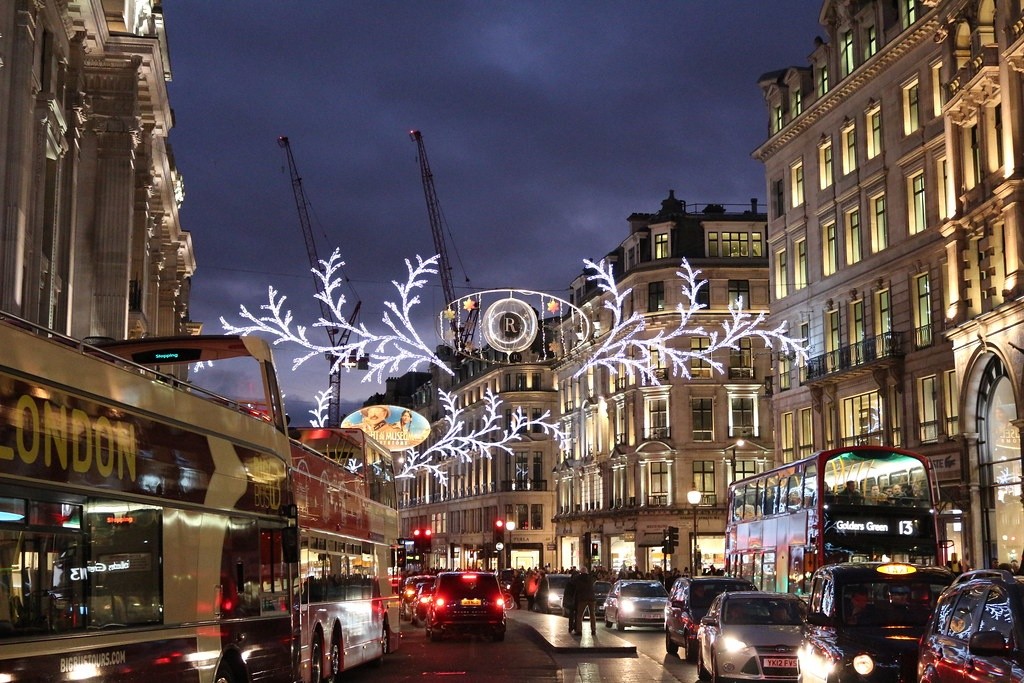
[503,593,515,610]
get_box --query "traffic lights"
[424,529,431,544]
[493,520,504,551]
[669,526,679,546]
[414,528,422,548]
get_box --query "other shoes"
[592,630,595,635]
[574,631,582,636]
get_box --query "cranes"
[277,129,481,427]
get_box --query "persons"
[353,405,412,439]
[406,567,727,636]
[845,553,1019,624]
[734,481,920,518]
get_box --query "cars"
[603,579,670,631]
[500,567,527,597]
[534,574,613,617]
[696,589,809,683]
[796,561,958,683]
[401,575,436,624]
[663,576,767,661]
[425,571,507,641]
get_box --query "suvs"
[916,569,1024,683]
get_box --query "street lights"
[731,440,744,481]
[687,491,702,576]
[507,522,515,549]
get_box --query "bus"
[0,309,407,683]
[724,446,959,611]
